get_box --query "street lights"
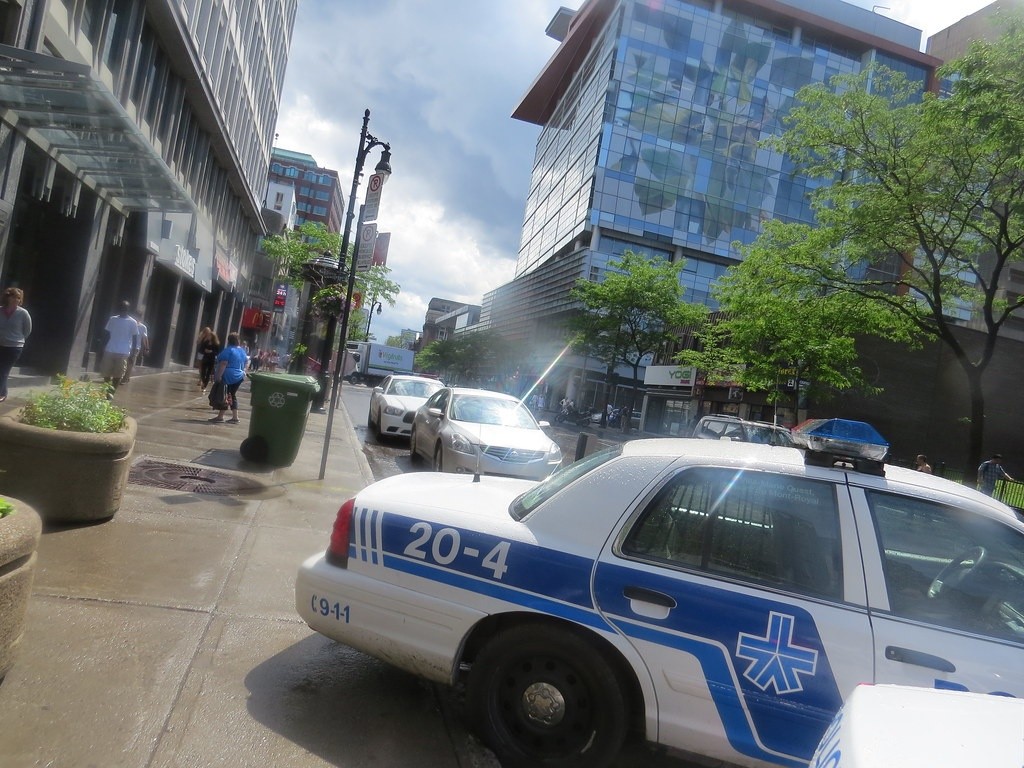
[364,298,383,342]
[308,107,392,414]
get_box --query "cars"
[292,409,1023,768]
[590,408,620,424]
[628,411,642,429]
[367,370,447,445]
[409,387,565,481]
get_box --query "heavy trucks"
[337,339,414,388]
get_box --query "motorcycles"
[556,403,595,428]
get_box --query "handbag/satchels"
[207,381,230,410]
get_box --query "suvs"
[690,414,799,451]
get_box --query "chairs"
[628,493,713,570]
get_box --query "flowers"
[309,285,356,324]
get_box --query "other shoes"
[0,395,7,402]
[226,419,240,424]
[208,417,225,423]
[120,378,129,385]
[202,389,207,392]
[107,389,114,400]
[198,381,202,386]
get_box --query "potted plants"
[0,495,43,679]
[0,373,137,522]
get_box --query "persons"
[622,405,630,416]
[241,340,277,373]
[916,455,931,474]
[560,397,574,413]
[99,300,139,399]
[531,393,543,413]
[209,332,249,423]
[122,310,148,383]
[196,328,220,391]
[607,403,613,420]
[978,454,1014,497]
[0,288,32,401]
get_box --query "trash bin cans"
[240,369,320,466]
